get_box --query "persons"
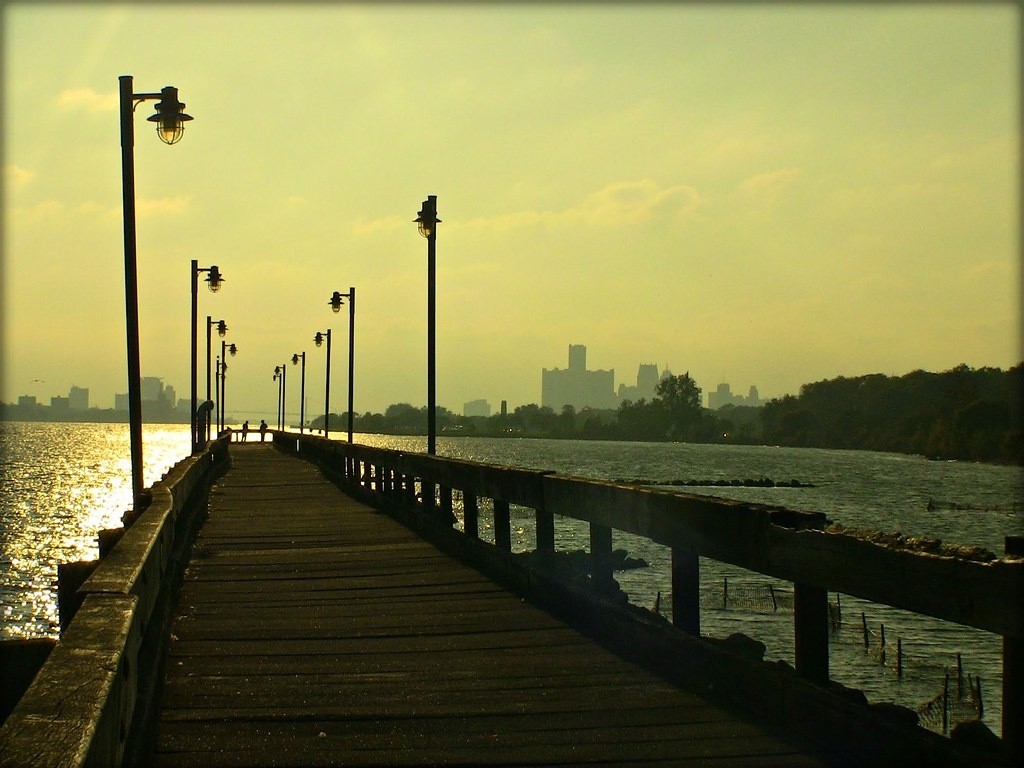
[260,420,268,442]
[241,421,248,442]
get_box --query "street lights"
[215,355,228,435]
[191,258,226,455]
[290,352,306,435]
[273,373,282,431]
[412,194,442,456]
[328,286,356,444]
[207,315,230,442]
[274,364,286,432]
[119,74,195,511]
[314,329,331,438]
[222,340,239,433]
